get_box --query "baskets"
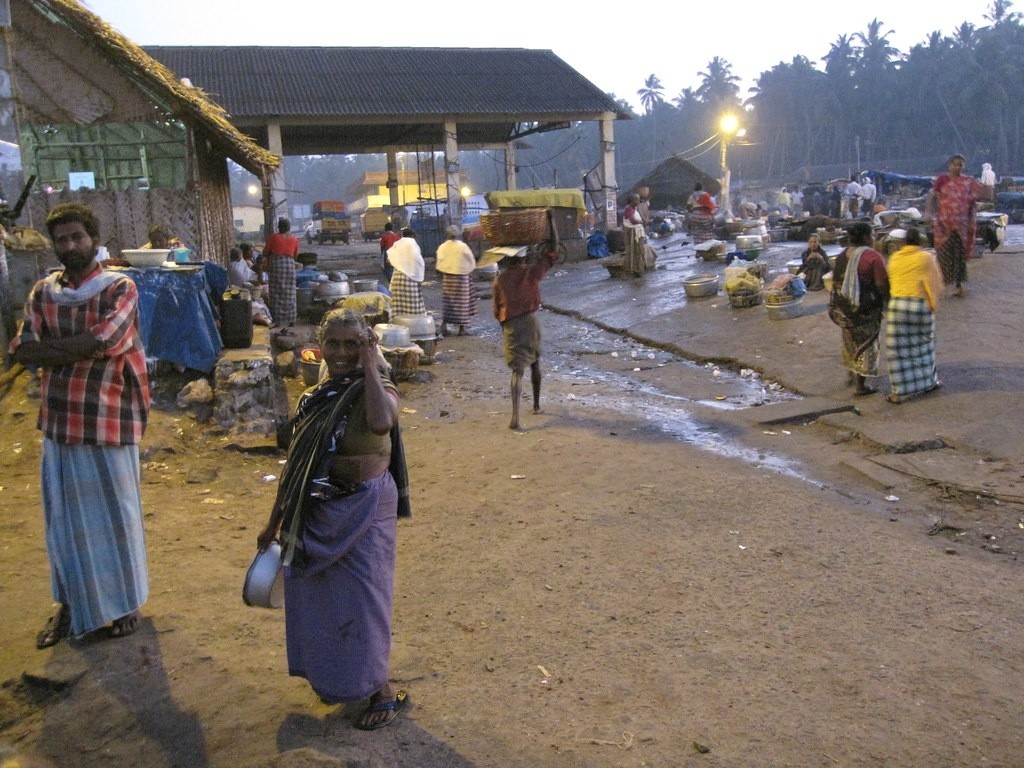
[478,208,546,244]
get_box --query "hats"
[447,224,459,235]
[517,247,526,257]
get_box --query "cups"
[172,243,191,263]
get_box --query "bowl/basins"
[683,274,719,296]
[786,258,804,275]
[736,225,769,252]
[727,288,764,307]
[314,282,350,301]
[301,349,321,361]
[373,323,414,348]
[121,249,172,267]
[478,263,501,273]
[762,296,805,319]
[393,314,437,340]
[353,278,379,293]
[242,537,285,608]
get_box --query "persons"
[829,223,889,396]
[305,223,314,243]
[386,229,427,315]
[380,223,401,282]
[687,182,716,246]
[231,243,268,298]
[10,205,149,647]
[263,219,299,324]
[257,308,411,730]
[981,162,995,185]
[436,225,479,335]
[926,154,984,297]
[738,201,761,220]
[494,208,559,428]
[885,228,942,402]
[845,175,887,219]
[623,193,657,276]
[802,234,830,291]
[778,186,841,218]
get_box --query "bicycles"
[526,235,568,266]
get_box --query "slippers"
[35,615,71,648]
[885,394,901,404]
[356,689,408,729]
[934,379,943,388]
[854,384,875,396]
[110,610,141,638]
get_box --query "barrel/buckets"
[296,287,316,315]
[300,358,322,386]
[221,290,253,348]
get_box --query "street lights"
[718,114,746,169]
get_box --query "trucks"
[306,218,349,245]
[360,212,393,242]
